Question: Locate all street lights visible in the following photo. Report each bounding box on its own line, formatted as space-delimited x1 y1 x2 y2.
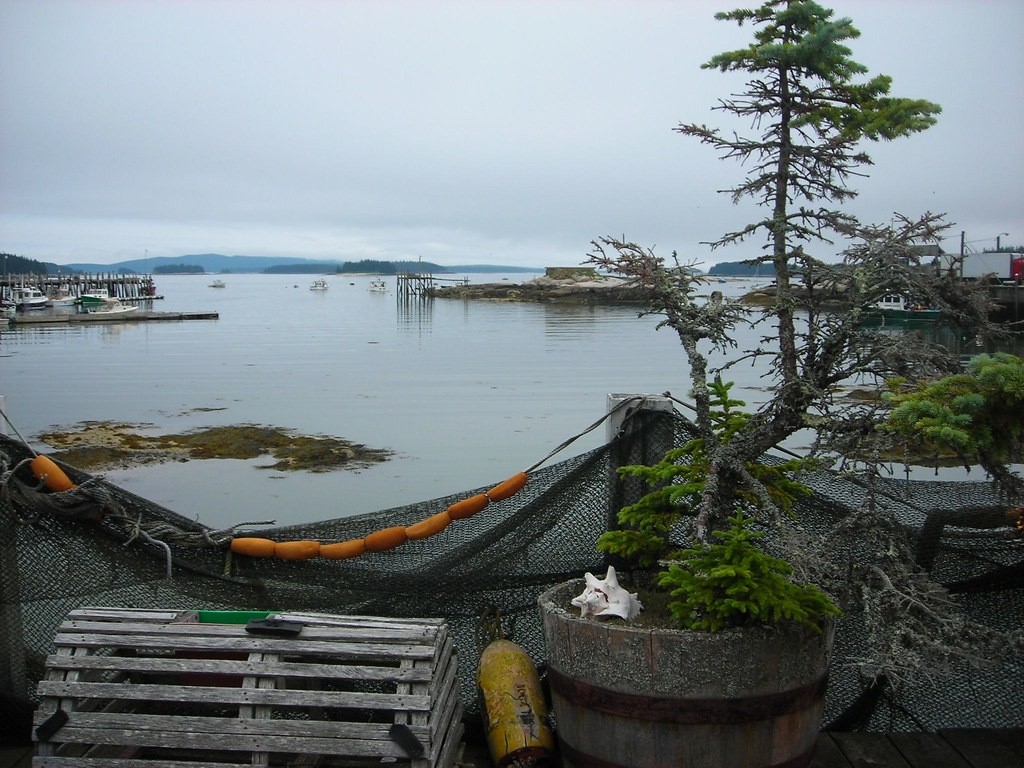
997 232 1010 251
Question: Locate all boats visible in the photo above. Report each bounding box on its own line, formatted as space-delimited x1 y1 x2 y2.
207 279 226 287
369 280 390 292
310 278 330 289
13 278 156 315
859 294 941 322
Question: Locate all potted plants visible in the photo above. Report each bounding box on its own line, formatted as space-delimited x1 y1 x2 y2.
881 355 1024 583
538 1 977 768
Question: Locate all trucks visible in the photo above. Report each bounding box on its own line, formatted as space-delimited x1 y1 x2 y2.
940 251 1024 282
